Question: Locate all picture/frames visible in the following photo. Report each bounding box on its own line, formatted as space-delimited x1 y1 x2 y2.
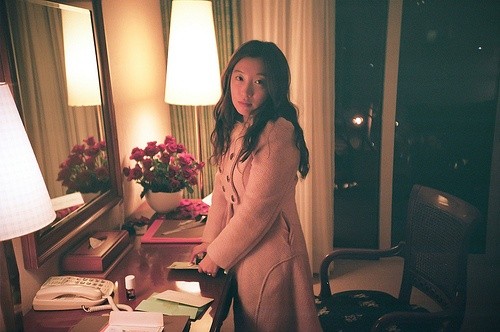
140 215 206 244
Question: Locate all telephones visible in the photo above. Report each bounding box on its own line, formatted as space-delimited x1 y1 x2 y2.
32 274 115 312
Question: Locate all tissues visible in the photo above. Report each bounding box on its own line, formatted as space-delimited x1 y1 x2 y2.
63 230 129 272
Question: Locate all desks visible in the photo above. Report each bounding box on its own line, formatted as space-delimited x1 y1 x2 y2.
23 230 232 332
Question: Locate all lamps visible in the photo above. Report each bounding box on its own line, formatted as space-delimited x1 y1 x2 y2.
164 0 223 200
61 8 104 142
0 82 56 332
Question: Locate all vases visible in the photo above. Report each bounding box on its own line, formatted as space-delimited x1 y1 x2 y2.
145 189 183 214
81 192 100 203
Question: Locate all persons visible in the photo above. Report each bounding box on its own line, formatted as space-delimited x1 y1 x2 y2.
190 41 322 332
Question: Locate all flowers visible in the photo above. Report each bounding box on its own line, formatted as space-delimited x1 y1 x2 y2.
55 136 109 194
123 136 205 200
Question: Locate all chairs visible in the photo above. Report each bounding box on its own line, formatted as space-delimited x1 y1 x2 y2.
314 183 482 332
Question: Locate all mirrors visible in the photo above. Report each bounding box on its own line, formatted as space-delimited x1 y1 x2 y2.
0 0 122 271
